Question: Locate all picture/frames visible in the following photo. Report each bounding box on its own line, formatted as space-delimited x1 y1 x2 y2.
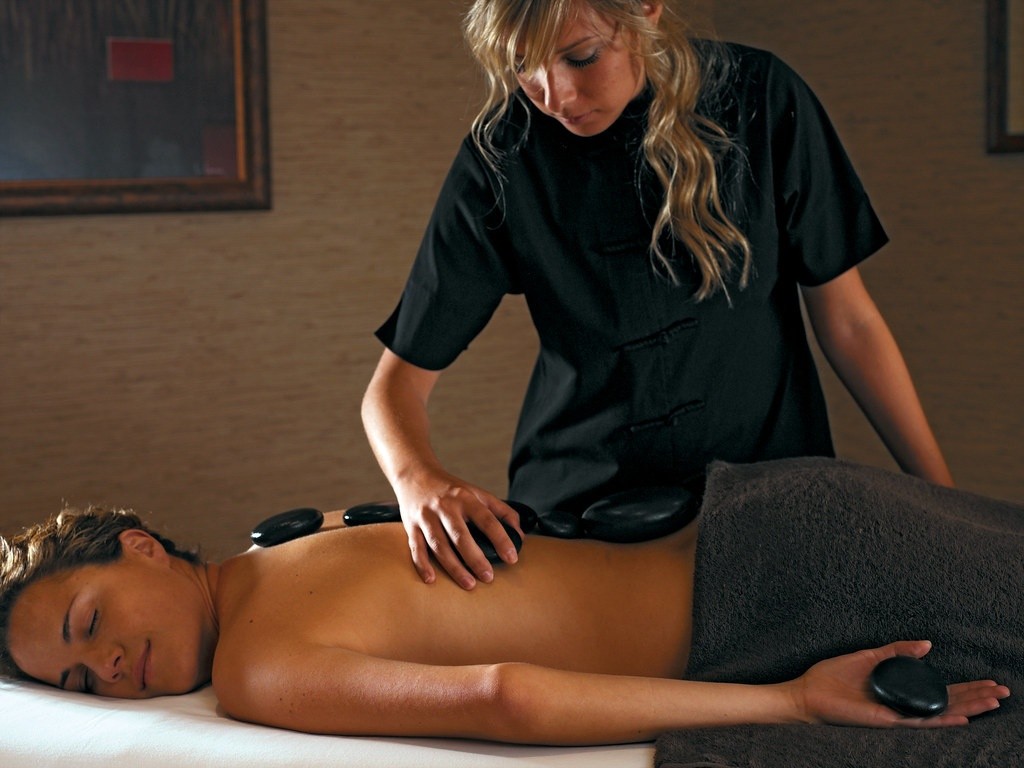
984 0 1024 157
0 0 272 217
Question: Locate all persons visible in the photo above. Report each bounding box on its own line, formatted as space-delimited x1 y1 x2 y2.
360 1 952 589
0 458 1024 747
